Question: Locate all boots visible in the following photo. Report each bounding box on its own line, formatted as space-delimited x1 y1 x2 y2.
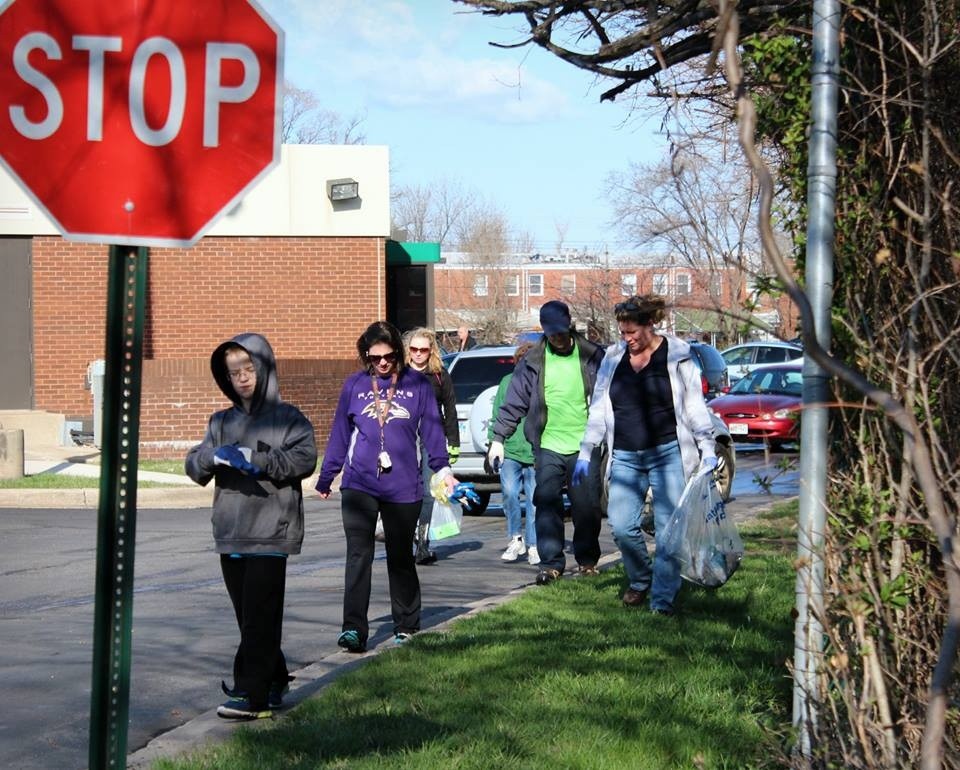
416 523 436 564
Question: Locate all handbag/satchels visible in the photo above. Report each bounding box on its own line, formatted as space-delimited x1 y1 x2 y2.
660 465 744 586
429 467 463 540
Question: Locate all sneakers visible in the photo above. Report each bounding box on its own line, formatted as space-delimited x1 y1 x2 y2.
536 567 562 584
501 534 527 562
395 630 416 644
579 564 596 577
268 693 284 708
528 546 541 566
216 695 274 719
336 630 367 649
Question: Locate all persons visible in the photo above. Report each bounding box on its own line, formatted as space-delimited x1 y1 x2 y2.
488 299 605 584
400 326 461 565
315 321 462 654
484 342 544 565
185 331 318 719
572 292 720 619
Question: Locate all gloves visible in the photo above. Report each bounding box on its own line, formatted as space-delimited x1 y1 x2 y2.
234 447 252 475
701 458 718 469
213 445 260 475
484 443 499 476
448 446 460 465
447 482 481 510
571 460 589 486
488 442 504 468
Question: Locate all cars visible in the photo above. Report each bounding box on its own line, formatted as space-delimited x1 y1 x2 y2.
706 365 804 450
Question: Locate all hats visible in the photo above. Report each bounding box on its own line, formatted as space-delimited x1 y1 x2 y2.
540 301 570 337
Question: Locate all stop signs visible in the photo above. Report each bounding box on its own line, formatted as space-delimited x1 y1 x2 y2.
0 0 285 248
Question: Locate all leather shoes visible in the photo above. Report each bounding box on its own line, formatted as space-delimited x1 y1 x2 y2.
623 587 647 606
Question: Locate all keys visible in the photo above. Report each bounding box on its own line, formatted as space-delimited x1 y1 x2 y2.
376 459 383 480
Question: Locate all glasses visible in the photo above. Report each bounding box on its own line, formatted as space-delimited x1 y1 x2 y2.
410 347 432 353
366 350 399 365
227 366 256 381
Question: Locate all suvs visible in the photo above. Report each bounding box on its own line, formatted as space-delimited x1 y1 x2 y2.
441 345 736 524
687 339 730 402
720 342 805 378
